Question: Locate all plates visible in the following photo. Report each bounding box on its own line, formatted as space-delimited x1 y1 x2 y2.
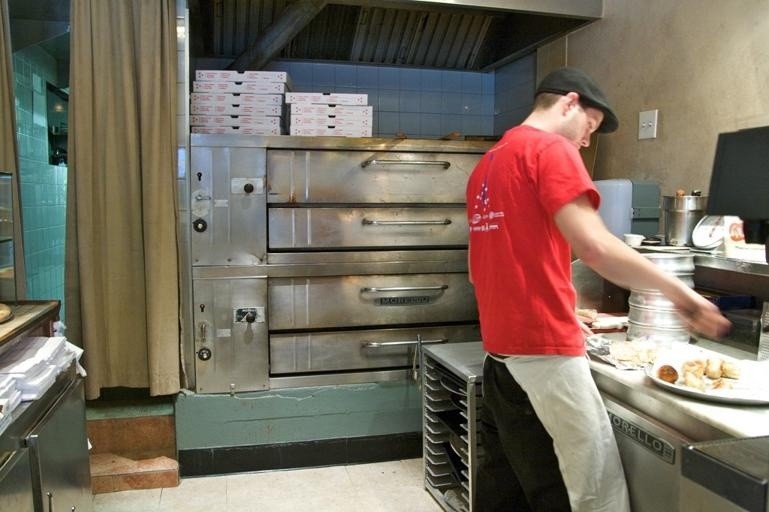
644 351 768 406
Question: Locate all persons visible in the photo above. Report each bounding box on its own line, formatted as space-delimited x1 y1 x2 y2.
466 67 731 512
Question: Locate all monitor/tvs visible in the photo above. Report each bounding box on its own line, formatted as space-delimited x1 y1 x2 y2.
706 126 769 222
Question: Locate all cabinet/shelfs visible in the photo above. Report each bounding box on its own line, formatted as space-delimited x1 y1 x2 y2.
678 436 769 512
0 297 93 512
422 339 484 512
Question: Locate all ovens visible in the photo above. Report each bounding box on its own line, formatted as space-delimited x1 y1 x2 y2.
190 270 486 394
191 133 504 268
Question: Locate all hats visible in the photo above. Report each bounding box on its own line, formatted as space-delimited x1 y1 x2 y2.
533 67 618 134
